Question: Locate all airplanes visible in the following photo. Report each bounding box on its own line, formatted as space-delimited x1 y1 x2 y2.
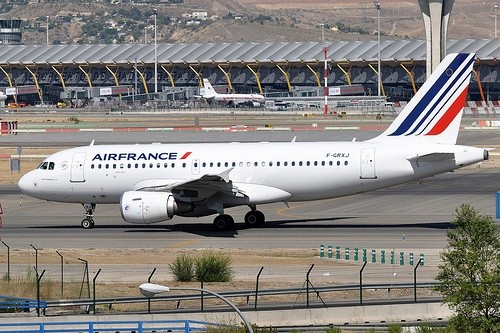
200 76 266 108
19 53 488 231
